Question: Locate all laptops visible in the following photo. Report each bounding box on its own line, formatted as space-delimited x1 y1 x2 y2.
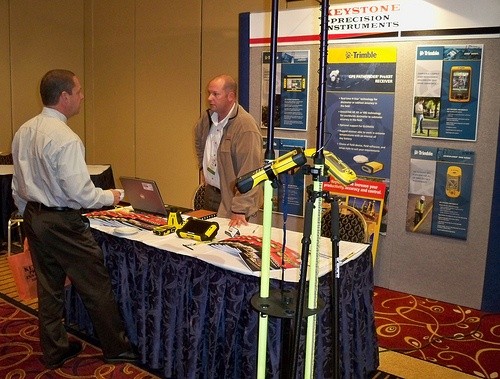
119 176 193 217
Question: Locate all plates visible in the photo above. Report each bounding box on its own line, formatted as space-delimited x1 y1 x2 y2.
115 227 139 235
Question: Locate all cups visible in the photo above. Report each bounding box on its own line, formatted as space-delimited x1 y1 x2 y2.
116 189 124 200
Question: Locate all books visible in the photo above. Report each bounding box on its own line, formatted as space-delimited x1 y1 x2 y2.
186 209 217 220
84 211 168 233
209 235 308 272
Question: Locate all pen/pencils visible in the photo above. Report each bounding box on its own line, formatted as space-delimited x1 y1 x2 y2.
342 253 354 263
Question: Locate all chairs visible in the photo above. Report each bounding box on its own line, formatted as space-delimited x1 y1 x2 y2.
318 205 368 245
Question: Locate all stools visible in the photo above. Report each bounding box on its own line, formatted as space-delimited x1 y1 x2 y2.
8 211 24 258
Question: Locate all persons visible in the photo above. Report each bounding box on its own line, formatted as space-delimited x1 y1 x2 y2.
415 99 425 134
12 70 143 368
194 74 263 228
453 76 465 88
415 196 425 225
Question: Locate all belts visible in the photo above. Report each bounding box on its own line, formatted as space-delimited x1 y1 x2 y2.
209 184 221 193
27 201 73 212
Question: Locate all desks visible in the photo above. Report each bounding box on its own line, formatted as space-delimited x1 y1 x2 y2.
0 164 112 246
65 206 380 379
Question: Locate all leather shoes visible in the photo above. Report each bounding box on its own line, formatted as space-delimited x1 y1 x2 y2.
104 346 140 362
46 341 82 367
